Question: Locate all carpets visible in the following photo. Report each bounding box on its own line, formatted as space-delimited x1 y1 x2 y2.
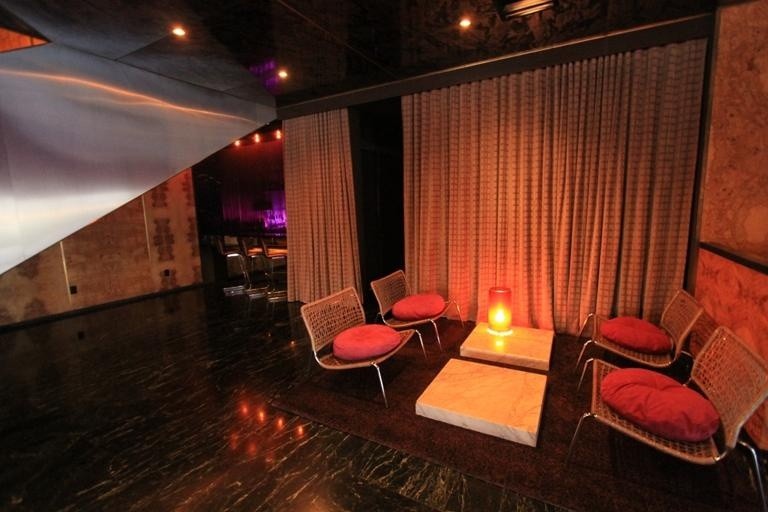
270 318 767 512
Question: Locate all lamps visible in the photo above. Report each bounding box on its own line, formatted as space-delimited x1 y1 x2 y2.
487 287 512 334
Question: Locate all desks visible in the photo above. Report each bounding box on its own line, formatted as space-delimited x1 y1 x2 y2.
415 322 554 448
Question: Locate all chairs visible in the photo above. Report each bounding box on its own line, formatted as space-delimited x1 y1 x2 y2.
212 237 287 303
300 270 465 408
